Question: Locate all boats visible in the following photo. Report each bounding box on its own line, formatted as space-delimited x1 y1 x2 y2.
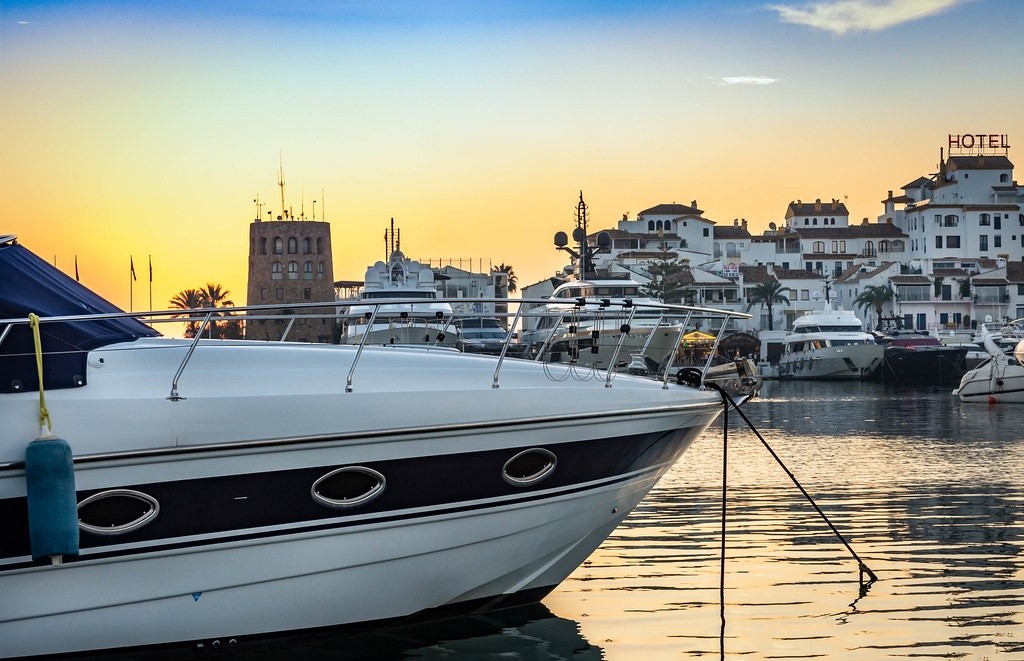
667 330 756 388
952 289 1024 405
522 189 683 374
0 236 748 661
970 290 1020 351
877 309 970 384
752 264 885 382
338 218 459 352
453 315 513 352
